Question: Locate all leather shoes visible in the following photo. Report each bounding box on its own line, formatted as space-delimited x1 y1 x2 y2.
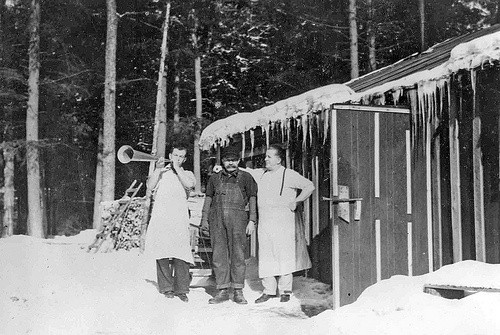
208 290 229 304
280 294 289 302
234 290 248 304
164 291 174 298
177 294 189 302
255 295 276 303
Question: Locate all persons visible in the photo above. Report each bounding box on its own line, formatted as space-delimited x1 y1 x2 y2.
213 143 315 303
145 146 197 302
201 146 258 304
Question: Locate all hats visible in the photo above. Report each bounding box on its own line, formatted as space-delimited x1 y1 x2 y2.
221 146 240 159
